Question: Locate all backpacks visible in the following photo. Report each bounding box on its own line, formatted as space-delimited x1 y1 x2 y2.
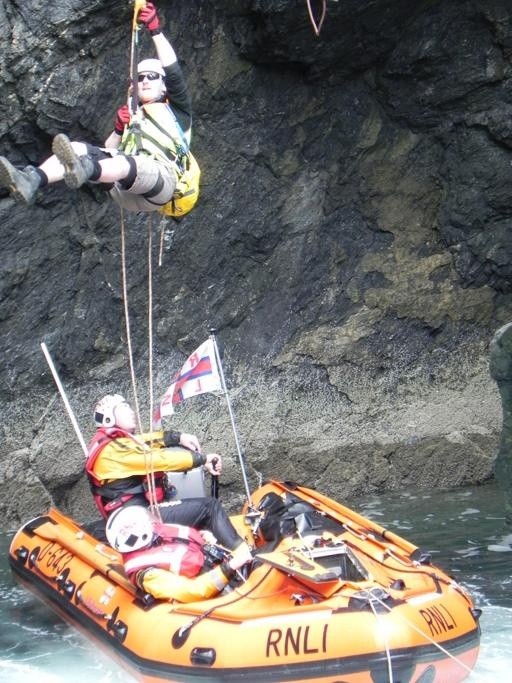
157 150 200 216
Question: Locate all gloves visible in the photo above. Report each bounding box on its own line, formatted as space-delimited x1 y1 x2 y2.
137 3 161 36
114 106 131 135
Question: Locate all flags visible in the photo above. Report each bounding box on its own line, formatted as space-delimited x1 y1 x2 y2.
153 336 218 422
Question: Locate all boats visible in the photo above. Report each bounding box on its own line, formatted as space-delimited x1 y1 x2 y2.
9 477 482 683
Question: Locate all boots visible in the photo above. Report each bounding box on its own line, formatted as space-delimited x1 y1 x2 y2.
1 156 41 205
51 134 94 189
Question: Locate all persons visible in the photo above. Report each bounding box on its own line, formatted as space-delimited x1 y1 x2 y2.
0 1 194 212
104 503 255 606
82 391 250 550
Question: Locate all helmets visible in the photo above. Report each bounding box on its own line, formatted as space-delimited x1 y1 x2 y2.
137 59 166 76
94 394 129 427
106 504 154 554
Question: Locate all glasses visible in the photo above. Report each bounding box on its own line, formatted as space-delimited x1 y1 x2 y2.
139 72 160 82
